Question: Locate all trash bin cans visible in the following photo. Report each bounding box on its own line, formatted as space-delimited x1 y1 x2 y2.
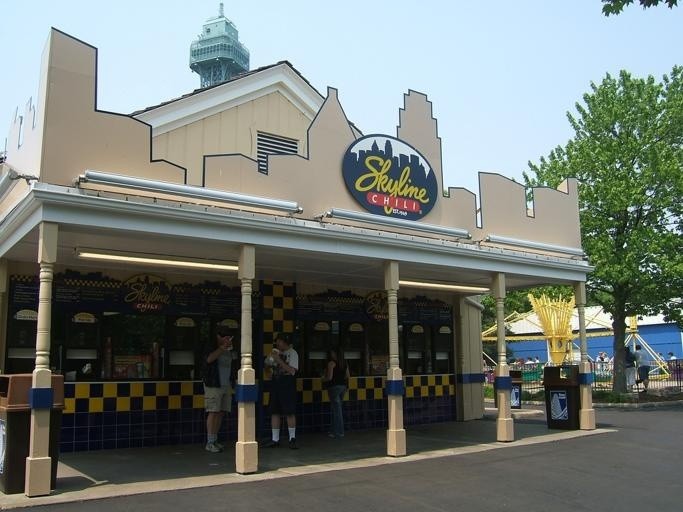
0 374 65 495
544 365 581 430
509 370 522 409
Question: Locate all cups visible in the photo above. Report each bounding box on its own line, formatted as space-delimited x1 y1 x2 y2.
136 362 144 378
270 348 279 361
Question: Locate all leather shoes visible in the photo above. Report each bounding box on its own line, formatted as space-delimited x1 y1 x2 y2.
262 440 280 449
205 441 224 452
288 438 298 450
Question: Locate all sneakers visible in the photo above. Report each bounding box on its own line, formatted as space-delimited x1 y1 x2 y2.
328 433 345 439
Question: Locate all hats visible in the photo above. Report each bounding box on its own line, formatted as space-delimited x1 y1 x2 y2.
215 326 233 336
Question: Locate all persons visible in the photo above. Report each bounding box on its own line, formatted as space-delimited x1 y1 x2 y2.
625 345 650 394
260 332 299 449
525 356 540 364
658 351 677 360
597 352 609 362
323 345 351 439
199 325 238 453
